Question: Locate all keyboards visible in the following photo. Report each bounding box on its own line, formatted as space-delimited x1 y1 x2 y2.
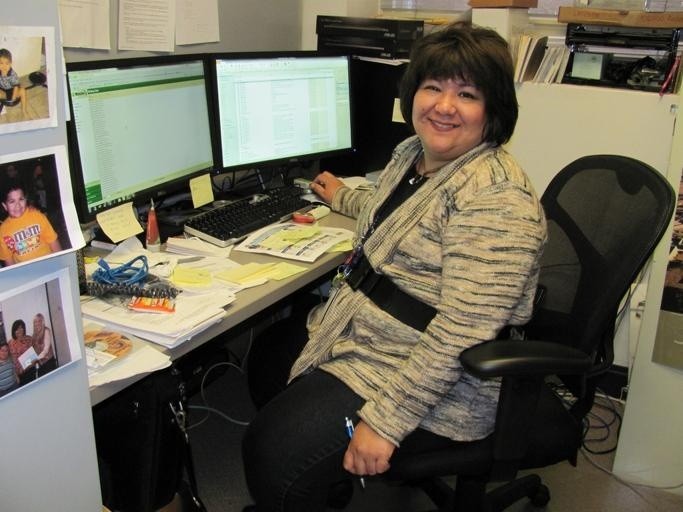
183 185 313 248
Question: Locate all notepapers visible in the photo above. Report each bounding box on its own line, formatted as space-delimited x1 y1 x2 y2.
95 201 145 243
189 173 214 209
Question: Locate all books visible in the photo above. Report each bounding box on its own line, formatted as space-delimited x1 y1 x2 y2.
508 32 570 84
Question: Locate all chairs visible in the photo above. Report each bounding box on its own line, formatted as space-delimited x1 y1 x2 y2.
357 155 675 508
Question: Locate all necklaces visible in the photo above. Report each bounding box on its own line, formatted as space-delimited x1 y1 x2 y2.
332 150 448 288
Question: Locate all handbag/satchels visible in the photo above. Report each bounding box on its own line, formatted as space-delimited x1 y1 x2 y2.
92 365 205 512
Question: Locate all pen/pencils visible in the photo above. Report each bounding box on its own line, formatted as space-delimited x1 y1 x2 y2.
344 416 367 491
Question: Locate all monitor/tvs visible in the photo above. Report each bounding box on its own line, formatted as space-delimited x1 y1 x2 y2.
210 49 357 174
66 53 220 223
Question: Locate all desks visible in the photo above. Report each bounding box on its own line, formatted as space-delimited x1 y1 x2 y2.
43 167 434 407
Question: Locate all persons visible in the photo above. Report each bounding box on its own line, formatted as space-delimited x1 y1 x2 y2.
0 48 28 116
31 313 57 378
0 343 20 400
0 185 62 267
8 319 36 386
240 21 548 511
